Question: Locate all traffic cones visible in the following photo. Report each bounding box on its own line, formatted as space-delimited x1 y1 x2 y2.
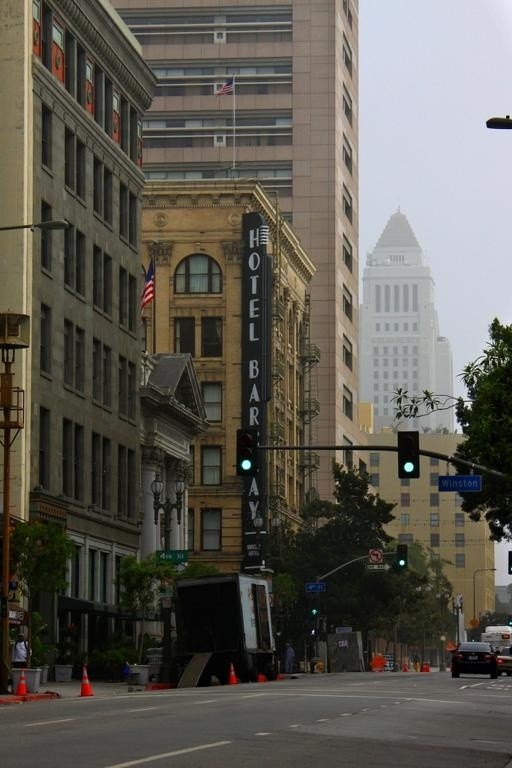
229 662 237 684
81 667 93 696
15 670 27 695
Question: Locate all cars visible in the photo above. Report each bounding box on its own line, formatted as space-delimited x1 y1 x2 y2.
452 641 498 679
497 645 512 676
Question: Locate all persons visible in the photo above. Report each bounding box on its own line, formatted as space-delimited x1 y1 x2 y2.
10 633 29 669
283 642 296 674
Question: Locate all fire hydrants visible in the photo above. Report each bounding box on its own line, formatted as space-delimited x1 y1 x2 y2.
414 661 419 671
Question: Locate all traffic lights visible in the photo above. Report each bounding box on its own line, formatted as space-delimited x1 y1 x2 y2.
397 544 407 568
235 428 259 477
308 607 318 636
398 430 421 480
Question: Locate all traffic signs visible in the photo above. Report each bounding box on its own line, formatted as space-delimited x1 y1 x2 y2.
305 583 326 592
438 475 482 492
156 549 187 563
365 562 391 573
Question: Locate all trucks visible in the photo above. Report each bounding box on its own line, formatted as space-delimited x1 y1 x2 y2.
481 626 512 648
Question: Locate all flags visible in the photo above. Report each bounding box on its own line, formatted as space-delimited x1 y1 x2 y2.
140 259 154 315
214 77 233 97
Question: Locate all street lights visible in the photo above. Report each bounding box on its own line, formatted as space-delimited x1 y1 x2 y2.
150 472 186 679
473 567 496 620
452 593 463 646
0 309 30 694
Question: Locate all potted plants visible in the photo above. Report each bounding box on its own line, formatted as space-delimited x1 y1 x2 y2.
10 516 80 695
114 551 179 687
